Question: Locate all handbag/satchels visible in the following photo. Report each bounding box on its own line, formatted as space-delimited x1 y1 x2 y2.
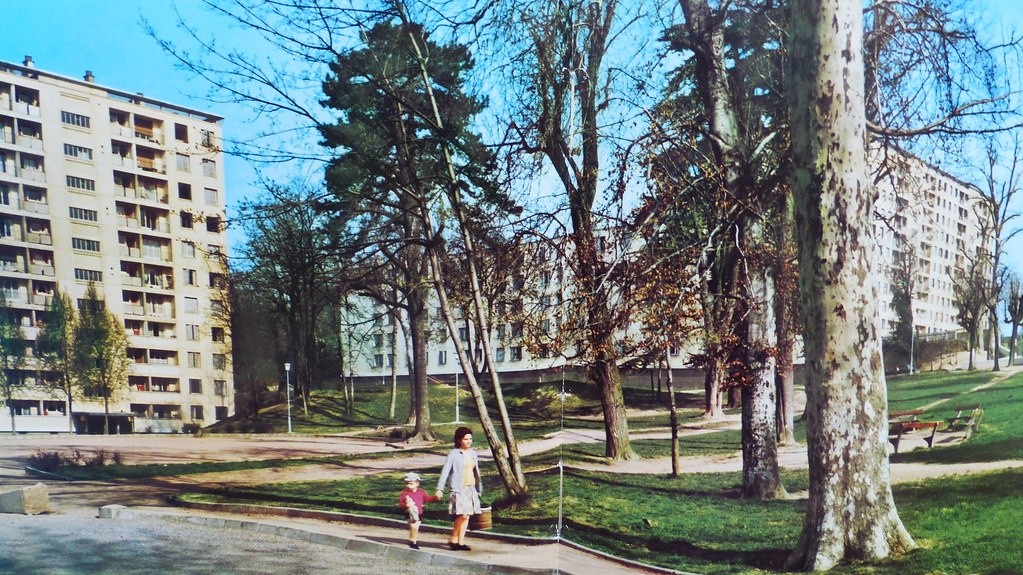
468 495 492 530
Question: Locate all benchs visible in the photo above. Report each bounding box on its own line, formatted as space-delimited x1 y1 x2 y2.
888 402 982 455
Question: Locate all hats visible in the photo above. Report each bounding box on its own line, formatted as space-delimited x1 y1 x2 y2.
404 473 421 481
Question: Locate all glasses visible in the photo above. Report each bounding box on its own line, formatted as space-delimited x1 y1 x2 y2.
405 482 414 485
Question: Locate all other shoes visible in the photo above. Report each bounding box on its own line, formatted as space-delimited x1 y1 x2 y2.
448 541 463 550
410 543 419 549
456 543 471 551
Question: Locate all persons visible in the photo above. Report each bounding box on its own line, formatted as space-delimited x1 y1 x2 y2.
398 473 440 550
436 426 483 551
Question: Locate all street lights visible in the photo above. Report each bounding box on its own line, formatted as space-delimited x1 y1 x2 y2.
453 350 461 420
284 363 292 431
